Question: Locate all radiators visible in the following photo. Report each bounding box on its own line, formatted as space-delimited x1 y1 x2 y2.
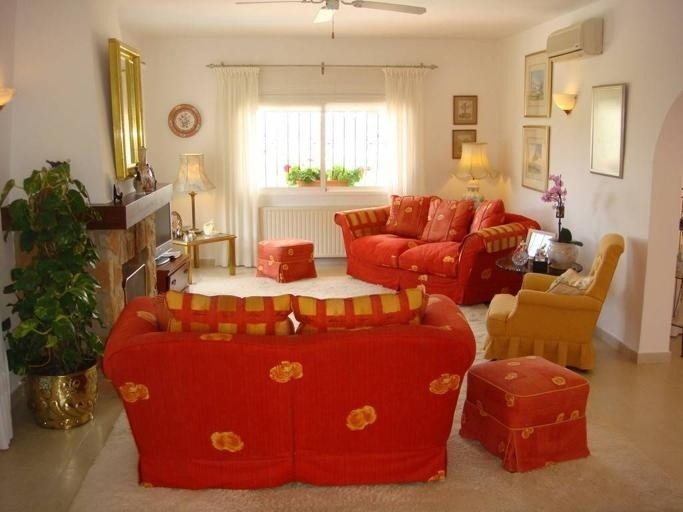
259 204 384 258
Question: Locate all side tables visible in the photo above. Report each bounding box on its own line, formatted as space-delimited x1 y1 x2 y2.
496 255 584 274
173 231 238 284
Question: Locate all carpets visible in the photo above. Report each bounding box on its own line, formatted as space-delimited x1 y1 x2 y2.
69 274 683 512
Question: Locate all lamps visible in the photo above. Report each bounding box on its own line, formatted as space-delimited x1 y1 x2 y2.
173 152 216 233
0 87 15 110
450 142 496 210
552 94 575 115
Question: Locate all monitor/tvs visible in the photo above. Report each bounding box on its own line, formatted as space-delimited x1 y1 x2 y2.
155 203 172 266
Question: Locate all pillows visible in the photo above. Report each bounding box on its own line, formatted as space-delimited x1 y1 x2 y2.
545 268 594 296
166 284 430 335
387 195 504 242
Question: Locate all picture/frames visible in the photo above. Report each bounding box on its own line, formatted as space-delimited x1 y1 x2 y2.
590 83 626 179
525 228 557 262
524 49 552 117
453 95 478 125
521 125 549 192
108 38 145 182
452 130 477 159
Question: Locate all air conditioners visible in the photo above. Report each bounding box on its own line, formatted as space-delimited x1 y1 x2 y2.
546 16 603 62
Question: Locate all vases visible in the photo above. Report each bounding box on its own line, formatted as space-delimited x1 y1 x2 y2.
556 206 564 218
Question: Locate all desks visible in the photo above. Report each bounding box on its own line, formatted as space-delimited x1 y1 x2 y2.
154 254 192 294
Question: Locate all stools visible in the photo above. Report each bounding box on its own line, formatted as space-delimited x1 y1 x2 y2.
459 356 591 472
256 239 318 284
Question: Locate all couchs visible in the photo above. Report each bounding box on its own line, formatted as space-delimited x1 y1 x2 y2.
101 296 477 489
334 207 540 305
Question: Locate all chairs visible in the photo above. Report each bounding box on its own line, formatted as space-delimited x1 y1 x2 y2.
483 233 624 371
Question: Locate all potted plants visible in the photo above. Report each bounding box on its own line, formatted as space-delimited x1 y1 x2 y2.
286 165 365 187
0 161 106 430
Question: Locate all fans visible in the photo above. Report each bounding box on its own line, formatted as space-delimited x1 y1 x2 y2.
235 0 428 23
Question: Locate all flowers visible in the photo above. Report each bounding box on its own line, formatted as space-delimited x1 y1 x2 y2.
539 173 568 209
